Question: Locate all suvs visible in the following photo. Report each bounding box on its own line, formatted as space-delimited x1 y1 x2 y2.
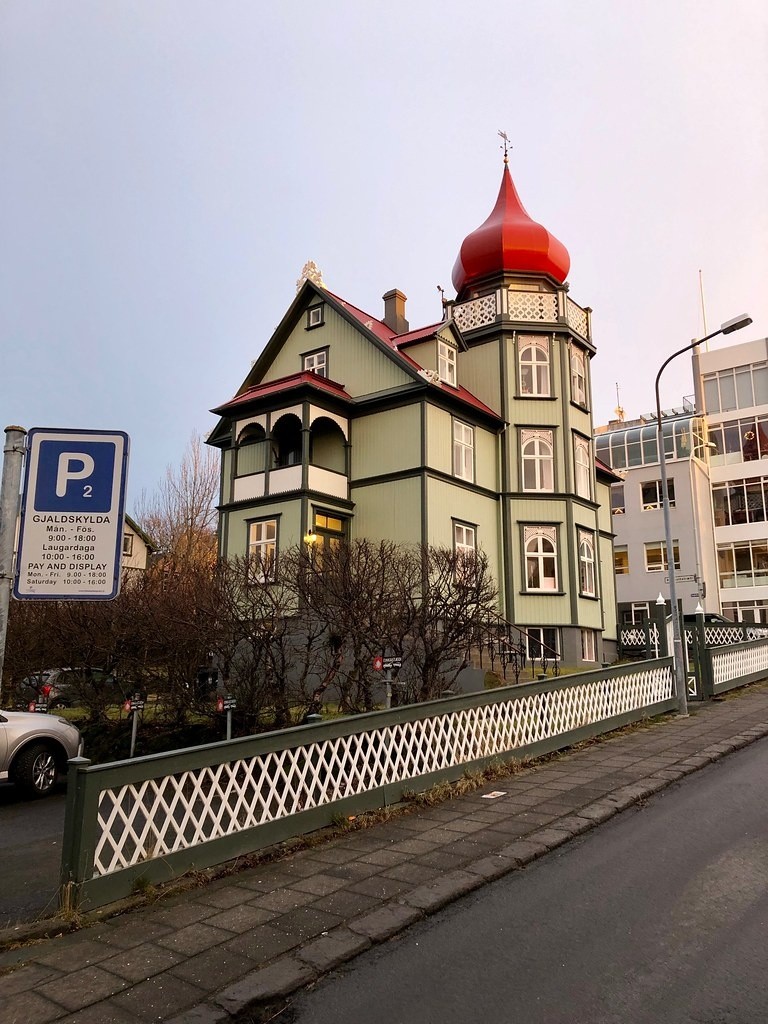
14 667 148 712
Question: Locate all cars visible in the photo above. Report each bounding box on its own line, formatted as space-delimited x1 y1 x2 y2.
0 709 84 797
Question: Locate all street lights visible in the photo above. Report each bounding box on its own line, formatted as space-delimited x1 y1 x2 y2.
654 314 754 717
688 439 716 611
